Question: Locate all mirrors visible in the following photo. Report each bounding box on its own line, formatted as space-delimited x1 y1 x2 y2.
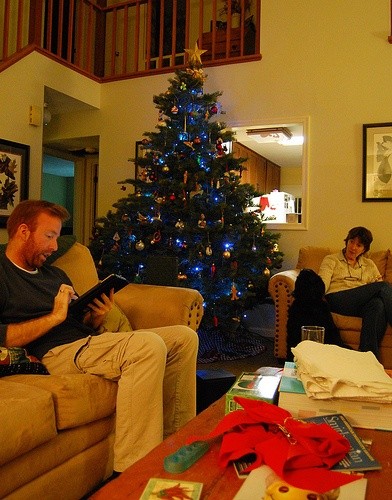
224 116 310 231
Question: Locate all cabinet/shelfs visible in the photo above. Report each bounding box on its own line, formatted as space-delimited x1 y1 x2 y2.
232 142 280 195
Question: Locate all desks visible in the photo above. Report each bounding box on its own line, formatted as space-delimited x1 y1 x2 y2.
88 365 392 500
195 26 253 61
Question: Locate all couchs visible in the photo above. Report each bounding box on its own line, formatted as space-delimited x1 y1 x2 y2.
267 246 392 371
0 238 203 500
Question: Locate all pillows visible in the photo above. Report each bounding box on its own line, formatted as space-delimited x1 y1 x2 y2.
0 347 50 378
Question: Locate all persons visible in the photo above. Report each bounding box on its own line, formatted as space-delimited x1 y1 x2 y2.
0 200 199 478
316 225 392 361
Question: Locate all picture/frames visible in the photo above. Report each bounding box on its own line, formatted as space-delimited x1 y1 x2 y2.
362 122 392 203
0 139 30 227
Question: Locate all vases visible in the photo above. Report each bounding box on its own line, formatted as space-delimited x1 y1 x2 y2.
230 13 241 28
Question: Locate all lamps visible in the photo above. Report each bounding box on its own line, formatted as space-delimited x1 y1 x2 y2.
44 101 52 126
244 127 292 145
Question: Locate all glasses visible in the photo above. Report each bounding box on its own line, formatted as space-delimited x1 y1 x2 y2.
344 276 358 281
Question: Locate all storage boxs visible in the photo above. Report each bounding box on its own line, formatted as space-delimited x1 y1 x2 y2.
278 370 392 431
225 371 282 415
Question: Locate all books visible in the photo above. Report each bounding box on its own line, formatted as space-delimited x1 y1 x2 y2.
277 361 392 432
232 414 382 480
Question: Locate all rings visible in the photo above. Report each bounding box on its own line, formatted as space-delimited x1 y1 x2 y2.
59 289 63 292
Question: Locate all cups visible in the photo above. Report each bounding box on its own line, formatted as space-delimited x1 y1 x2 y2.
301 326 325 345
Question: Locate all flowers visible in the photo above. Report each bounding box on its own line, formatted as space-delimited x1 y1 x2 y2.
219 0 251 19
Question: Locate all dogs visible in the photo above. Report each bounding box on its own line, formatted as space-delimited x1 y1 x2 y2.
284 266 352 362
